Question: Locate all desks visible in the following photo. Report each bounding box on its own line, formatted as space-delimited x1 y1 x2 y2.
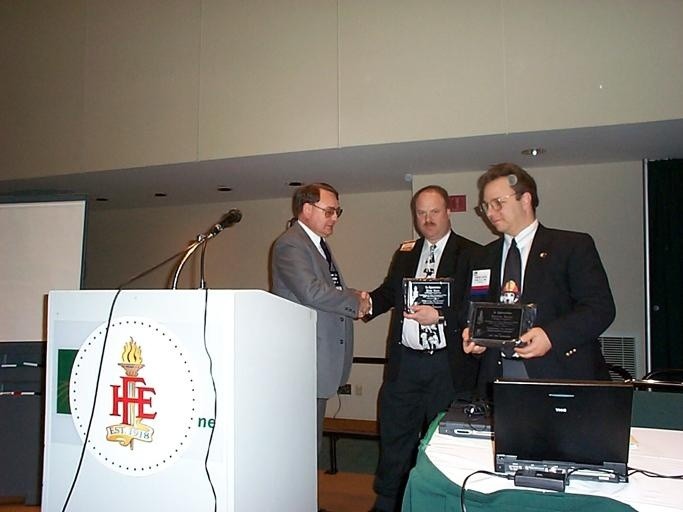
402 391 683 512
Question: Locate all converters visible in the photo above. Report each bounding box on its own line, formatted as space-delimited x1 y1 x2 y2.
514 469 566 492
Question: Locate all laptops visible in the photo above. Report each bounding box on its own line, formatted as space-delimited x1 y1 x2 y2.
439 399 494 438
493 377 634 483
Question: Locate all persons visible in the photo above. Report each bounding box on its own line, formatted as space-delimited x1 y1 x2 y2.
460 163 616 402
354 185 487 512
267 182 369 457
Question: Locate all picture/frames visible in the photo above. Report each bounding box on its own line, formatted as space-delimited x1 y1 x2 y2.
401 277 454 315
467 303 525 347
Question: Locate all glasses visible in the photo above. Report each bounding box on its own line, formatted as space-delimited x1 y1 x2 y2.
310 203 344 219
480 190 524 215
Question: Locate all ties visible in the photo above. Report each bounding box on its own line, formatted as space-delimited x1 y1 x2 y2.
421 244 437 279
502 237 521 290
320 237 342 291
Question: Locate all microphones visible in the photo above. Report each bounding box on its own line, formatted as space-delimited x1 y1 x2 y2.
210 209 241 236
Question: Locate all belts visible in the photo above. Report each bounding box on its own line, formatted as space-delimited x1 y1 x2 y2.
399 343 447 356
